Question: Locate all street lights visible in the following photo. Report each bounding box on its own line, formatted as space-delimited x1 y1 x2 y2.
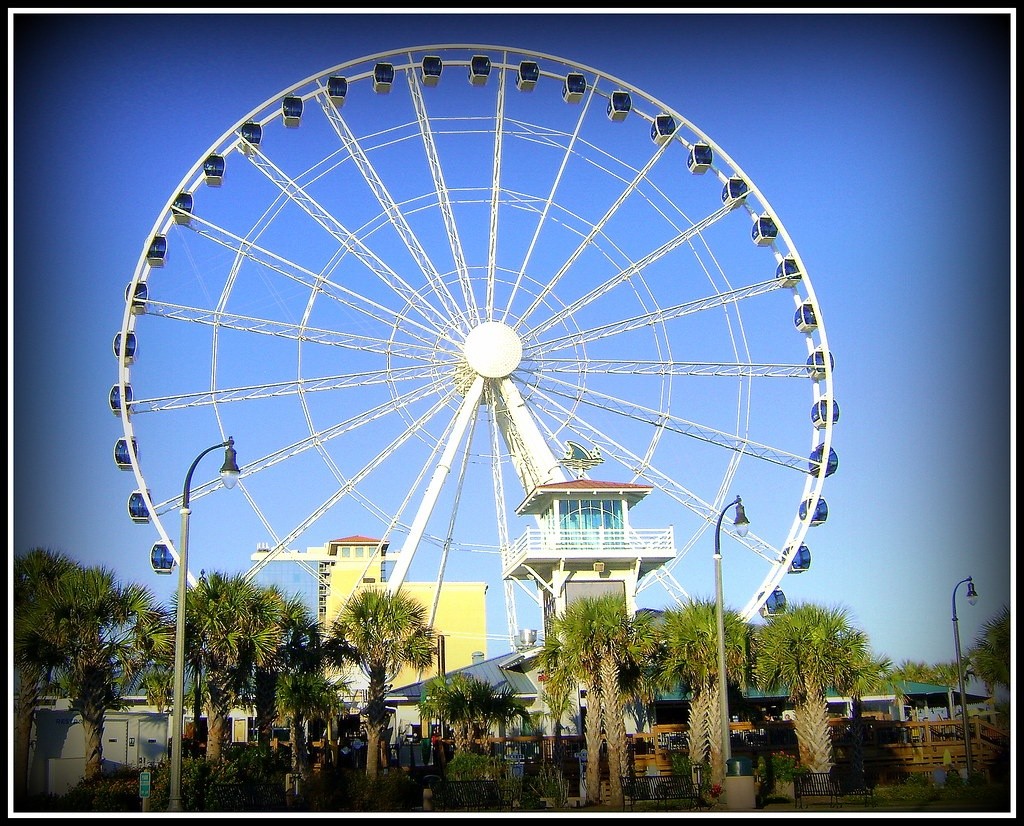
713 495 750 777
165 435 241 812
951 576 978 777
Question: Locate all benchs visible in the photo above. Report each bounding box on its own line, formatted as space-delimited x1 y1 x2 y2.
214 783 297 811
428 779 514 812
619 775 696 811
793 772 878 809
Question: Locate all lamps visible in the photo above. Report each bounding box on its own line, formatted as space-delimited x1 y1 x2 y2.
592 562 605 572
526 573 535 581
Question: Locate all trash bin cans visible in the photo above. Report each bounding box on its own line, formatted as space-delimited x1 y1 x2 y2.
285 774 302 793
725 755 753 776
985 759 1008 785
